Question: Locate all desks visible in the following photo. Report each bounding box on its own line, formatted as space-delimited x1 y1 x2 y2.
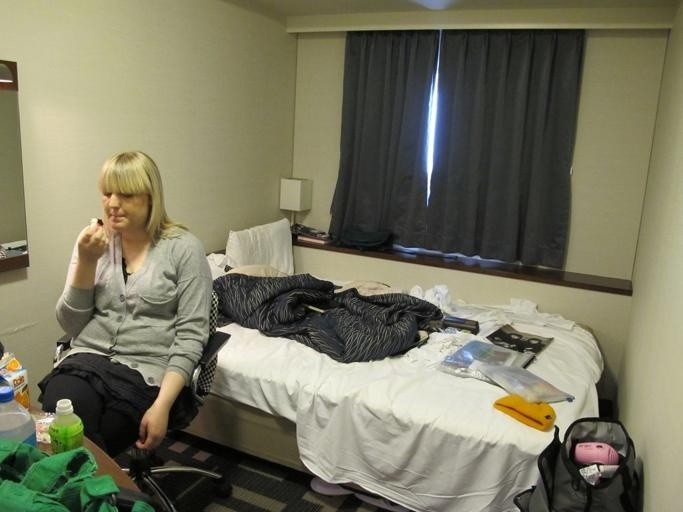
0 399 145 512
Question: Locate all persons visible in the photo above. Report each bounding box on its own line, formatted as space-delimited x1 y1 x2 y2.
492 332 523 351
32 151 212 461
514 337 543 354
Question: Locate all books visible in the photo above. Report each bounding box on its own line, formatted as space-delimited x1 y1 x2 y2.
296 234 336 245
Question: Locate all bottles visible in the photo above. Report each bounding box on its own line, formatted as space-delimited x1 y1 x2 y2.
0 386 36 451
47 398 86 455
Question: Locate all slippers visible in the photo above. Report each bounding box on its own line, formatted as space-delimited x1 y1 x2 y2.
356 492 410 511
310 477 354 497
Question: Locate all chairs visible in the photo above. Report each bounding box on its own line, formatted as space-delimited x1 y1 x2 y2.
52 288 231 512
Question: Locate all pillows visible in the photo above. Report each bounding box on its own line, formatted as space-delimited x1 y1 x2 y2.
220 216 293 287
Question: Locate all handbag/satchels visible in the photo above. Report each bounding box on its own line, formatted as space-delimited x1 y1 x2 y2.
335 229 395 252
1 441 154 512
512 417 638 512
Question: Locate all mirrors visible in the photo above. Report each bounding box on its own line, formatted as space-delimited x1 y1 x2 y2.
0 57 30 272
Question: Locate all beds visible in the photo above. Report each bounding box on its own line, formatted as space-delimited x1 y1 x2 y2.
137 242 603 512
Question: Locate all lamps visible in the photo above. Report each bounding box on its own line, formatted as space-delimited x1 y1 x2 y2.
279 177 313 244
